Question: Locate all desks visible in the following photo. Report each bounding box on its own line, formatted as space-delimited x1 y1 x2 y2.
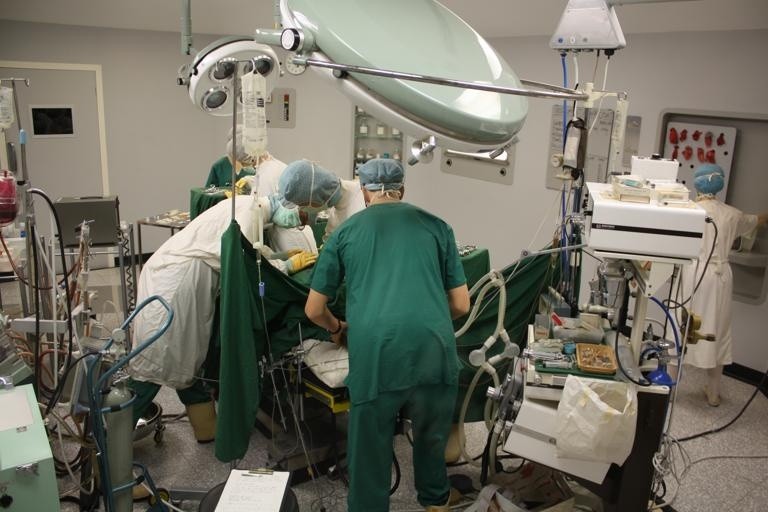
137 209 192 279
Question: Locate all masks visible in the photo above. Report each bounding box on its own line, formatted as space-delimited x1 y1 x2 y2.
301 204 329 215
272 201 302 228
241 164 256 174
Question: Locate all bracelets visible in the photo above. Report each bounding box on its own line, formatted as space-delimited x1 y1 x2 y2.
327 318 342 335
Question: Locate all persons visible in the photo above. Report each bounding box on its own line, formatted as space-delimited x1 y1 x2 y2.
204 124 255 192
683 161 768 407
225 132 287 200
304 156 475 511
275 159 368 252
120 190 320 445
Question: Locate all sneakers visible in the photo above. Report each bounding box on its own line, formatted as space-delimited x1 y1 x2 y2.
422 474 473 507
704 384 721 407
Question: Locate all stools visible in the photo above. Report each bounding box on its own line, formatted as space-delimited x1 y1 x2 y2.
193 477 300 512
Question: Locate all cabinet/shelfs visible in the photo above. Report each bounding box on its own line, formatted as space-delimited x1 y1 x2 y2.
346 101 403 188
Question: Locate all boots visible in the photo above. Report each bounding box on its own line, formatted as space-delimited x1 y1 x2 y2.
184 401 219 445
131 480 153 501
